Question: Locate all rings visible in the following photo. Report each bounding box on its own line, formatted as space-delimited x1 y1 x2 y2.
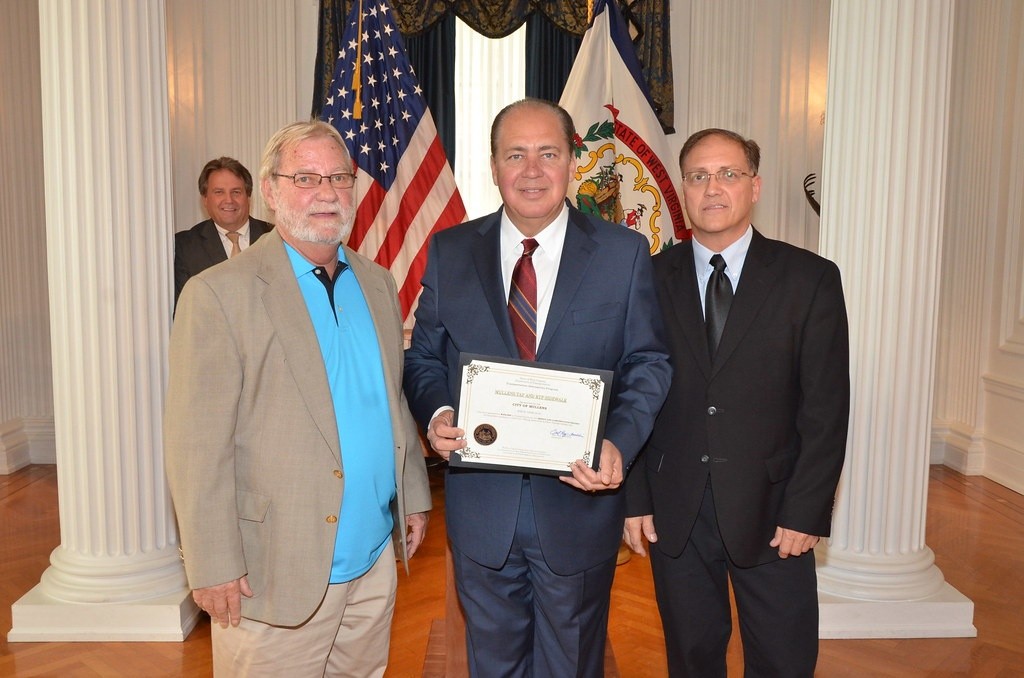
591 490 596 493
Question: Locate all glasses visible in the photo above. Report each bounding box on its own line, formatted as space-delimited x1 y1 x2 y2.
682 169 755 184
273 173 358 190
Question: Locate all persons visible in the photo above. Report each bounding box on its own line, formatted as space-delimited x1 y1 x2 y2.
163 121 432 678
172 156 276 323
403 99 673 678
622 128 850 678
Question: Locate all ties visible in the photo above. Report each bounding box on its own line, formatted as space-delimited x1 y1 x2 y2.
507 238 539 361
705 254 734 363
226 232 241 259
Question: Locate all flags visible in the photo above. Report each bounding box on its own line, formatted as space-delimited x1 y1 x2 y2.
320 0 469 330
558 0 693 256
